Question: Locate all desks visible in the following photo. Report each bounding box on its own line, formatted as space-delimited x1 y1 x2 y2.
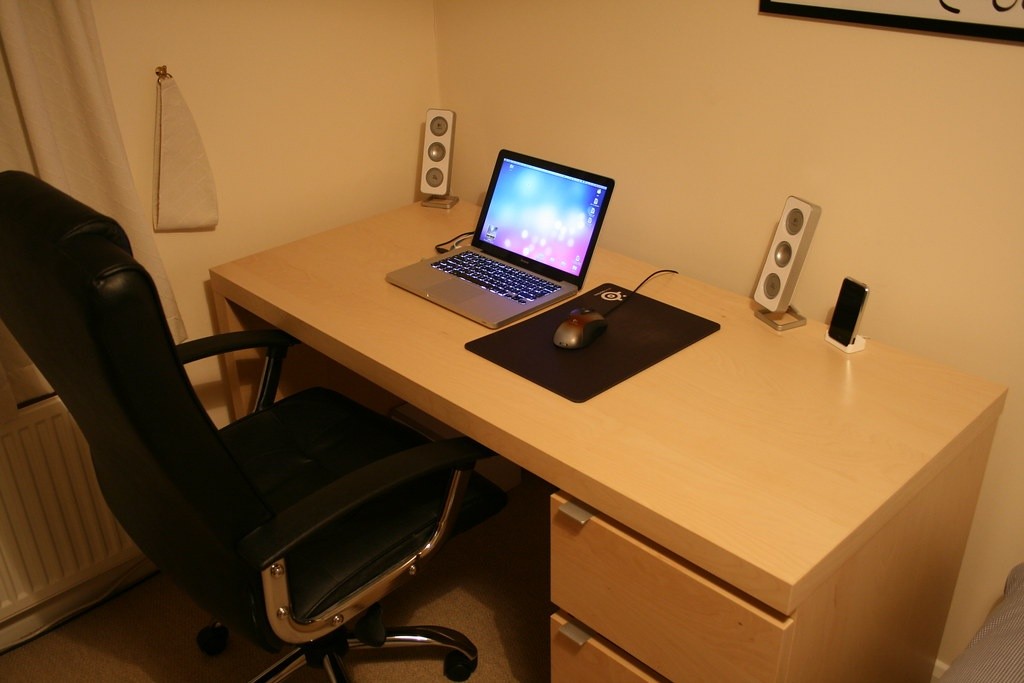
210 194 1007 683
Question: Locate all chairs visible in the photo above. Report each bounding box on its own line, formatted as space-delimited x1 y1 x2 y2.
0 169 510 682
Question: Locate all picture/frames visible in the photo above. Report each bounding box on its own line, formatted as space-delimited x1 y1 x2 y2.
757 0 1024 42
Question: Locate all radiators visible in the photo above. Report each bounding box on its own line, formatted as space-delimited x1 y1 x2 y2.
0 394 152 623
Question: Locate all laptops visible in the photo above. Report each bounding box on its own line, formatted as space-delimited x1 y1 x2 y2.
385 149 616 329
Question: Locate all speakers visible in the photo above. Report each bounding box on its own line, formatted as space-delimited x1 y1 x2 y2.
754 195 821 331
421 108 459 209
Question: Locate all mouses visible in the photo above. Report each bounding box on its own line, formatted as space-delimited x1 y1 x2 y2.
553 307 607 350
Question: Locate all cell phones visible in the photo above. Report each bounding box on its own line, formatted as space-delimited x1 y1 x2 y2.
828 276 870 347
